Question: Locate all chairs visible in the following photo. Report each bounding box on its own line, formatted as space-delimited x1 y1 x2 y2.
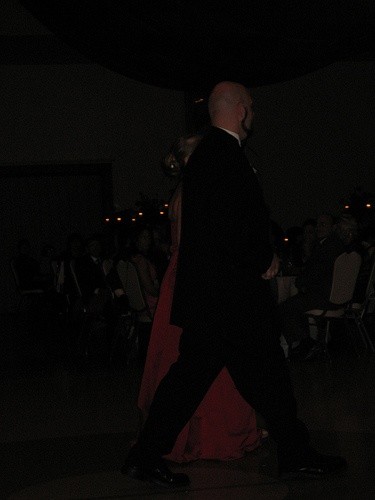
114 259 154 348
304 249 375 370
10 254 57 320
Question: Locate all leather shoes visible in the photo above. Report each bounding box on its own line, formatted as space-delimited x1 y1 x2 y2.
256 436 347 476
121 445 191 489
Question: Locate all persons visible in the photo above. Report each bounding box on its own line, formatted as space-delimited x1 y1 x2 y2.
135 125 271 478
10 208 375 366
120 80 347 489
